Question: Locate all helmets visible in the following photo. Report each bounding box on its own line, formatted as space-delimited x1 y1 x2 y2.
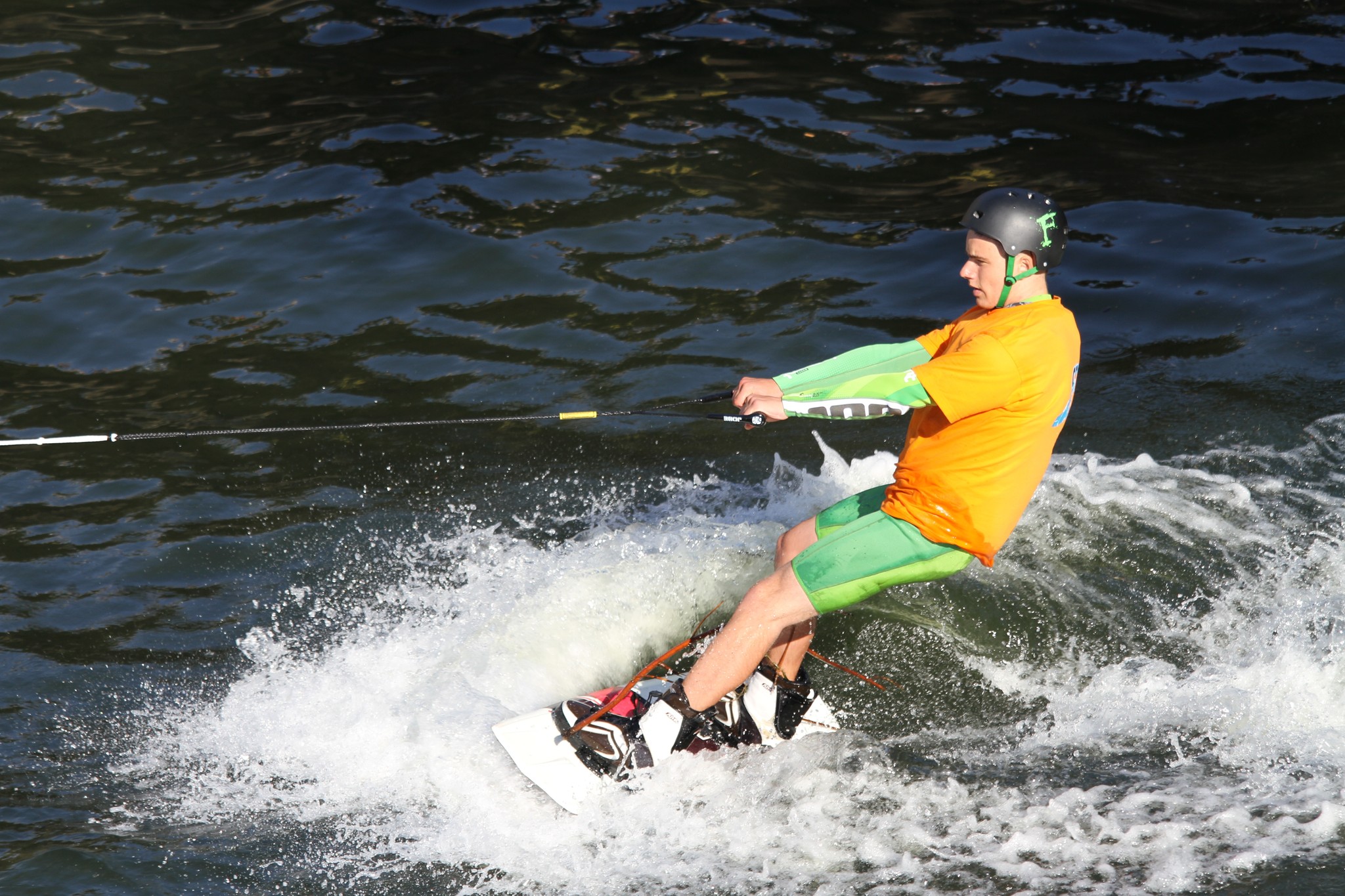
971 186 1067 271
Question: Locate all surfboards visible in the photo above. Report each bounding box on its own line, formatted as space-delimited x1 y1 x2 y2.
491 661 840 816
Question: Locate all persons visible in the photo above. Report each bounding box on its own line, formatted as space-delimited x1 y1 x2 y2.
556 187 1082 795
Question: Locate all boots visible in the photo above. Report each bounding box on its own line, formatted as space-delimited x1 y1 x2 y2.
556 678 720 780
711 655 816 749
